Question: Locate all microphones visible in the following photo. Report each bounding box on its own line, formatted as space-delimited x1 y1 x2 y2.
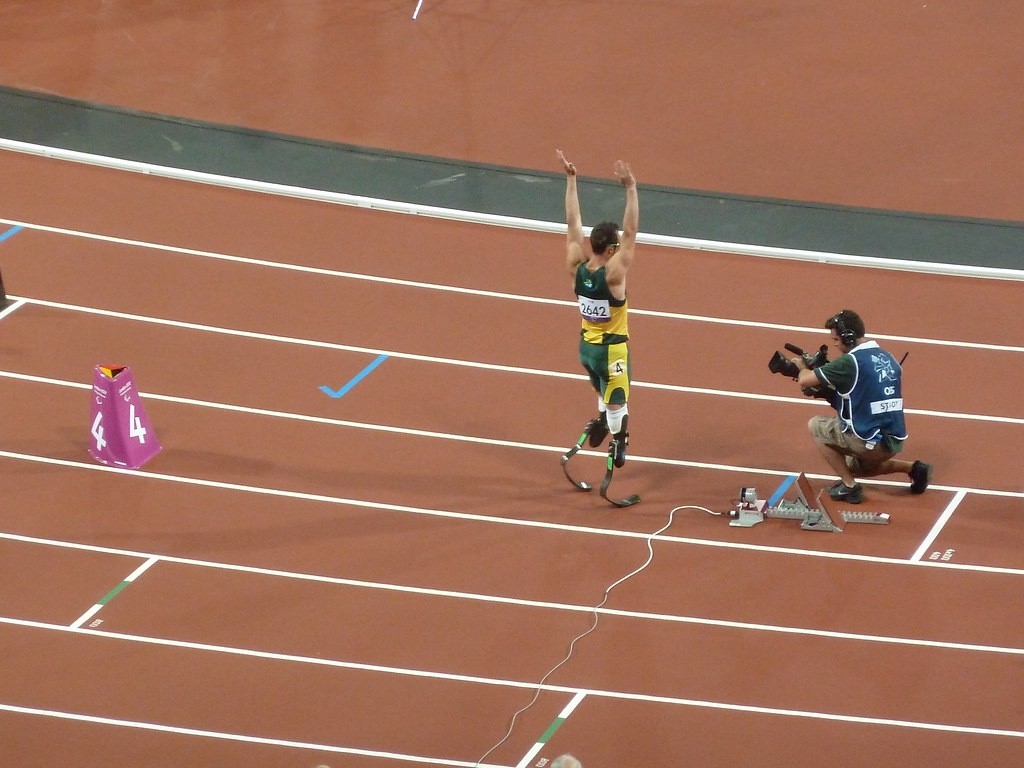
784 343 808 358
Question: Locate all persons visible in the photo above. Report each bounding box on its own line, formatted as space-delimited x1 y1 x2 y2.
790 309 934 506
555 148 639 468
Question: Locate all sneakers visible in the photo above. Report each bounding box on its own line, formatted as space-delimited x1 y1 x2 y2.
908 460 932 494
827 480 864 504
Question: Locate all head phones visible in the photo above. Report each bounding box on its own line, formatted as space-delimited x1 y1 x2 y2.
834 314 858 347
